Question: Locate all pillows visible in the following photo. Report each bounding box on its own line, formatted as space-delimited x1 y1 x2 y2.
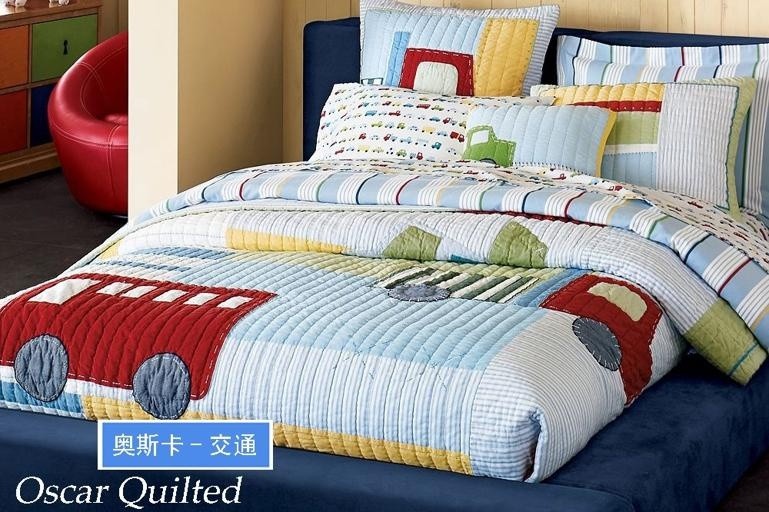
528 76 761 226
311 79 534 175
552 32 769 226
355 1 562 104
457 97 620 186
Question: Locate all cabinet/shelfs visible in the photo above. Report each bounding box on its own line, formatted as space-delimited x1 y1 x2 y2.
0 1 103 187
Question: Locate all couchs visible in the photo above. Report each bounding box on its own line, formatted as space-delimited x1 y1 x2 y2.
44 29 131 216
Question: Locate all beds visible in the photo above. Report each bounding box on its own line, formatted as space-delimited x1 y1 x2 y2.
0 1 769 512
